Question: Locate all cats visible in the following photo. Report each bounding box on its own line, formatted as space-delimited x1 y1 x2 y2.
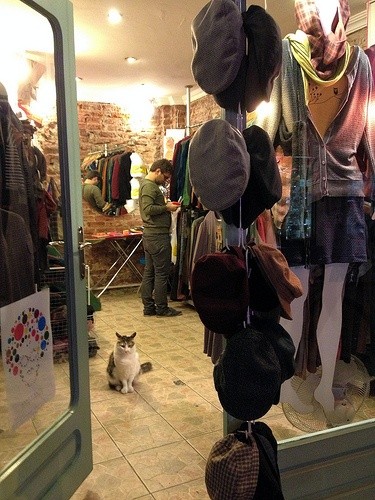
107 331 152 393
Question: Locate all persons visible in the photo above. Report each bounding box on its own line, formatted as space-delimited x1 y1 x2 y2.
82 170 112 213
137 158 183 316
265 32 375 415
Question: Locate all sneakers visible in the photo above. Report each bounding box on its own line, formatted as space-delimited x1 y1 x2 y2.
156 307 183 316
144 307 156 315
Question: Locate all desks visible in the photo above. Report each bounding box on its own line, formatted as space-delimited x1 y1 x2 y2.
86 230 143 298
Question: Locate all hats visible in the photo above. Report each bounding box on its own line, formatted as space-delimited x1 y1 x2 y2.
188 121 250 211
212 339 281 420
190 246 247 334
192 0 246 92
246 4 283 113
247 242 304 320
242 124 283 230
232 420 285 500
246 318 294 383
204 430 259 500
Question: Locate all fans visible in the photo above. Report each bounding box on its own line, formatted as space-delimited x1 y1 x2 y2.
282 356 370 433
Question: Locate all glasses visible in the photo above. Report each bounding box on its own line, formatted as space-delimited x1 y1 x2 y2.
161 170 169 181
